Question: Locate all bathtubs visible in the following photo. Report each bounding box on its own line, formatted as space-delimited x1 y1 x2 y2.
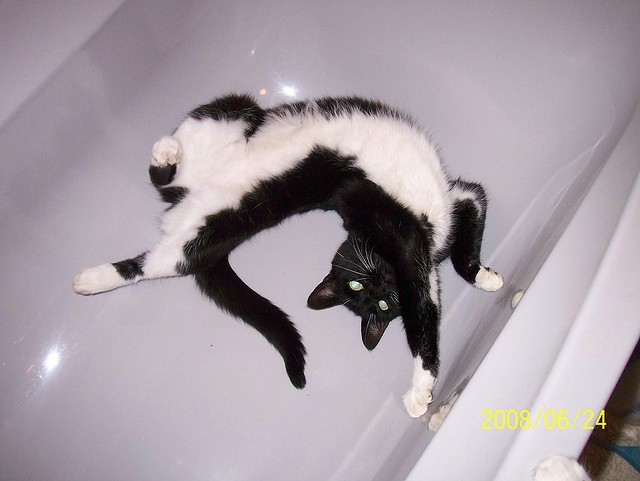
0 1 640 481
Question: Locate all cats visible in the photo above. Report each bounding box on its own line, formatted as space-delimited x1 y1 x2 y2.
72 92 503 418
